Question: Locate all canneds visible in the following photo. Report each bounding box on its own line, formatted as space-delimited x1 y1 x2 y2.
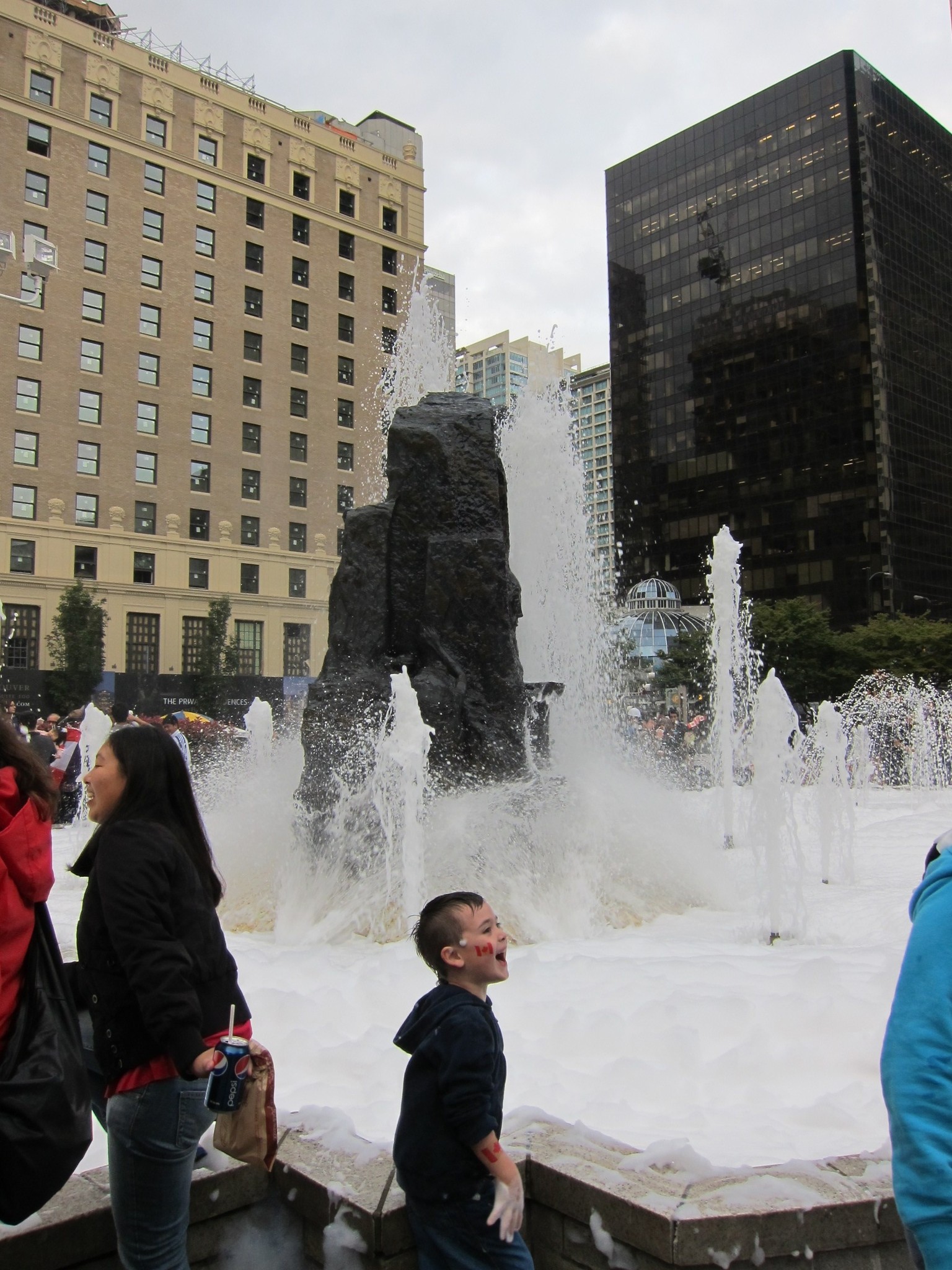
203 1036 249 1114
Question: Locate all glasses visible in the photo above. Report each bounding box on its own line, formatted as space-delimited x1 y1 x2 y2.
47 720 56 725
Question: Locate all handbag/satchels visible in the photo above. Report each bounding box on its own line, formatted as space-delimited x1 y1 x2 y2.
0 904 92 1225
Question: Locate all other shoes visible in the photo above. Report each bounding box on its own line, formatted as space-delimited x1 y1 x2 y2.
193 1144 208 1171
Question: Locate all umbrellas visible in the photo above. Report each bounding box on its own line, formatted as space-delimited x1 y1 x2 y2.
160 709 213 723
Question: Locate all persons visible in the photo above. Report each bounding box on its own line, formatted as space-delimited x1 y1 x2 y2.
626 700 705 757
0 699 64 761
392 892 536 1270
112 703 154 733
0 718 55 1038
64 726 272 1270
163 714 190 770
878 828 952 1270
